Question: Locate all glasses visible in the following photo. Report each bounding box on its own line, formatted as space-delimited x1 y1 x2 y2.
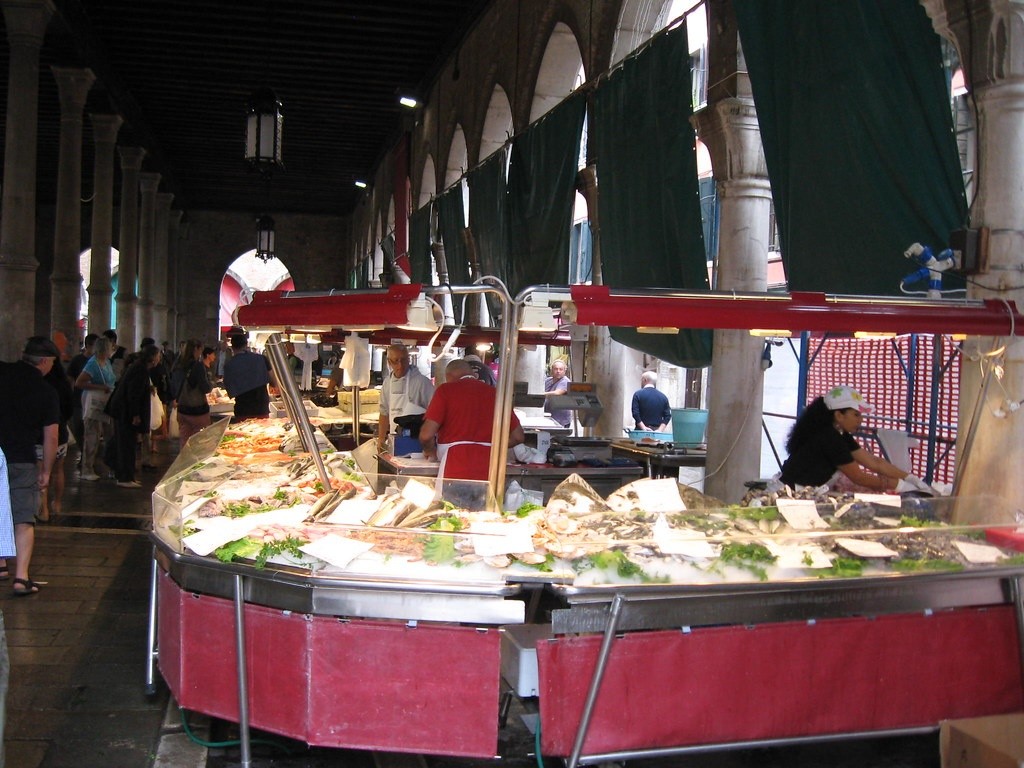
390 359 411 365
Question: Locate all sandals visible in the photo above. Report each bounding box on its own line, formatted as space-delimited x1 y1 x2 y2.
12 578 43 595
0 566 12 580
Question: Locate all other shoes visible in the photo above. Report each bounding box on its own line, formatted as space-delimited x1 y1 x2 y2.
80 472 101 481
116 479 142 488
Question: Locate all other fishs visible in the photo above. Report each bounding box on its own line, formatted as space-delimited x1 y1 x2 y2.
168 383 999 573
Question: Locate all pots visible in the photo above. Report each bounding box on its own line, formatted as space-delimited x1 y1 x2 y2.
623 428 672 442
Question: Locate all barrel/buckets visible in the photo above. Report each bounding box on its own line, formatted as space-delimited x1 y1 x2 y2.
671 408 708 448
395 435 437 456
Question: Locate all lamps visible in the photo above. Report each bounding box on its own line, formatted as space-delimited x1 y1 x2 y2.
395 90 425 109
290 334 306 343
636 326 680 334
397 293 439 332
306 334 322 344
342 324 385 332
351 174 370 189
749 329 792 338
854 332 897 341
247 326 286 333
255 182 277 261
243 0 286 184
291 325 332 333
281 334 289 342
517 305 559 333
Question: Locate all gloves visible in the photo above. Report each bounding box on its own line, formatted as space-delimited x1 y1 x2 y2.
895 474 928 493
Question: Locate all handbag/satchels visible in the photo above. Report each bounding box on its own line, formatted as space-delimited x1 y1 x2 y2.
150 390 164 429
168 408 180 438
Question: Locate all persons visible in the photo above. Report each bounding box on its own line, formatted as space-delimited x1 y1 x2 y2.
465 345 498 385
420 360 526 510
780 386 931 494
285 341 323 378
544 360 573 429
225 333 276 421
67 334 100 465
631 371 671 431
377 344 436 451
36 369 72 525
75 339 116 480
168 340 211 451
200 340 229 384
0 448 16 740
105 331 181 489
0 337 61 595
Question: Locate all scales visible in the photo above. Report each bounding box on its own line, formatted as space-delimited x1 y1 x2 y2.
552 382 612 461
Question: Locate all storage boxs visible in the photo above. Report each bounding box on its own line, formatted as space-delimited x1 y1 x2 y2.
269 400 319 418
206 388 235 412
337 389 380 415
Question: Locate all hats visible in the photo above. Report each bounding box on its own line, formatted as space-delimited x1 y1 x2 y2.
823 386 876 413
22 336 60 357
